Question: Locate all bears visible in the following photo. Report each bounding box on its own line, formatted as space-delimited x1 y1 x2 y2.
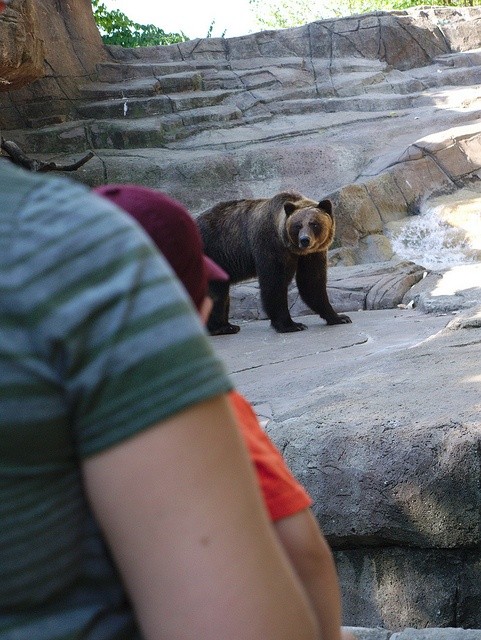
192 192 351 336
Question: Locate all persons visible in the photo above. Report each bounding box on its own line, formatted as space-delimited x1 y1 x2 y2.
83 182 360 639
0 149 327 639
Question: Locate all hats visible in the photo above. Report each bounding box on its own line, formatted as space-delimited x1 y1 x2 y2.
95 185 230 285
